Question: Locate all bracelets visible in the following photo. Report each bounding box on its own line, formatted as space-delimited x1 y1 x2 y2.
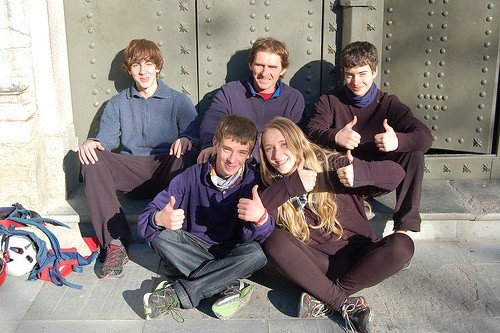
254 208 267 225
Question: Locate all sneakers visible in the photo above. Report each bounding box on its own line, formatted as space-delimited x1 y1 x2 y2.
100 243 129 279
337 296 373 333
211 279 256 320
143 280 184 323
297 292 336 319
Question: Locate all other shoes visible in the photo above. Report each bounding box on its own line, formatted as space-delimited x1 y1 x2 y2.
402 259 411 271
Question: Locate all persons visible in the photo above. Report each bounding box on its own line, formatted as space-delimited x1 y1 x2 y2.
78 39 202 280
304 41 433 271
200 37 306 189
136 114 275 320
258 117 415 333
